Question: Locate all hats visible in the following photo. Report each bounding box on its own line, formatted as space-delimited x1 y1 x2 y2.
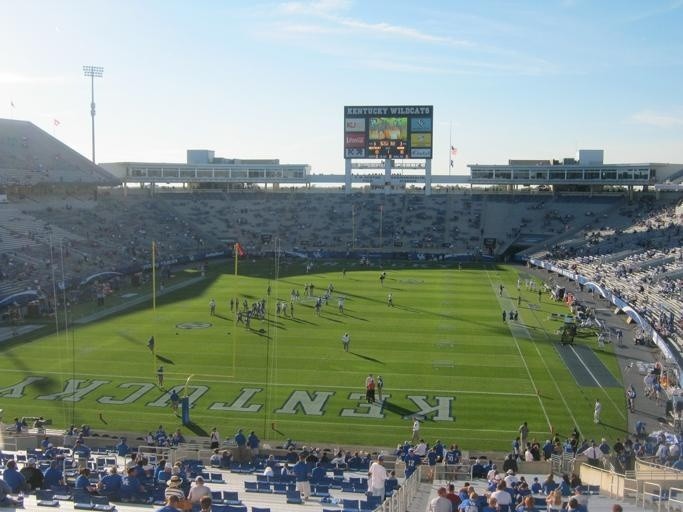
167 476 183 487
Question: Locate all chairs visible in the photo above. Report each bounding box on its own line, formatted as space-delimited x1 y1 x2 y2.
2 193 683 386
0 437 683 512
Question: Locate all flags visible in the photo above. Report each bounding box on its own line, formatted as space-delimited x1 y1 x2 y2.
451 160 454 167
451 145 457 156
54 119 60 127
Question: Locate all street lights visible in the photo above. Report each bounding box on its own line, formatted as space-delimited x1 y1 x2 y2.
83 65 103 201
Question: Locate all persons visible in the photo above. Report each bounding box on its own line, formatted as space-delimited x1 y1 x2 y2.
1 174 215 335
499 173 683 511
170 389 180 413
158 366 164 387
342 333 350 352
377 375 384 400
276 282 345 318
366 374 376 403
148 336 154 353
388 293 393 307
212 173 544 278
380 272 386 286
231 284 272 329
1 416 511 511
